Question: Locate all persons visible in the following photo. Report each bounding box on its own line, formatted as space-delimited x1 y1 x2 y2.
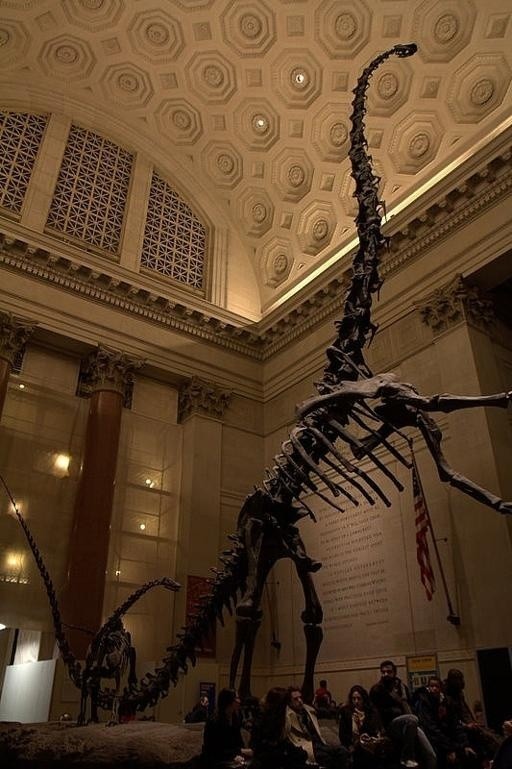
315 679 332 700
338 660 512 769
201 686 254 769
285 686 352 769
247 686 310 769
184 693 212 723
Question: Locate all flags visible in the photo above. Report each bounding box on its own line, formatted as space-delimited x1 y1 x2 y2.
409 456 438 602
